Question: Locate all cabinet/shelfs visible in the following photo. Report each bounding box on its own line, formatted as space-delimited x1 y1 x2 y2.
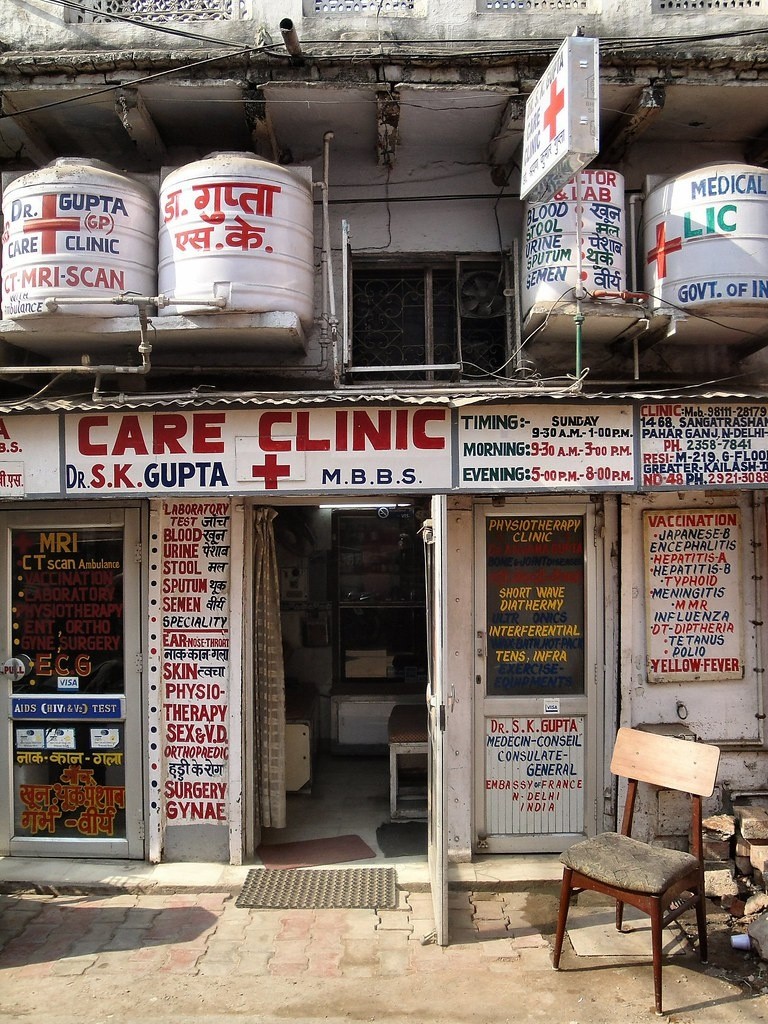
329 508 431 695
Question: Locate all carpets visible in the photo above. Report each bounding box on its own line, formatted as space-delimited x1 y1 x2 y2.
376 821 428 858
235 867 398 910
256 834 377 869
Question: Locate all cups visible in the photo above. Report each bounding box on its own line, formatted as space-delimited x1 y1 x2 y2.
730 934 750 950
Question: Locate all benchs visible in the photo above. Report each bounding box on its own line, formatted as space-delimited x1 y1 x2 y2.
282 679 318 795
387 704 429 821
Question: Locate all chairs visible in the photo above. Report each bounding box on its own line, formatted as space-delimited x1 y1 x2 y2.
552 726 720 1017
